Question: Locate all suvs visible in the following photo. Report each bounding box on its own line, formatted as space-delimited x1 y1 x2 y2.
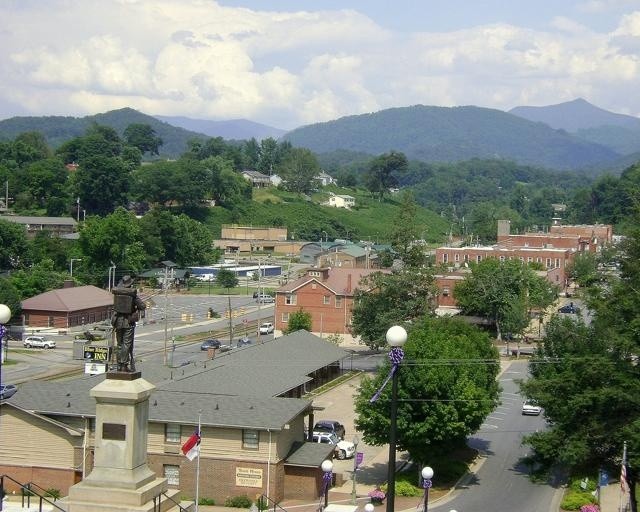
307 431 355 460
23 336 56 349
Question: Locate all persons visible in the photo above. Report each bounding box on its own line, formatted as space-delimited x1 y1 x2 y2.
112 275 146 372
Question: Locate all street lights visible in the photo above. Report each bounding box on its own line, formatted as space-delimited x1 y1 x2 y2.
365 503 374 512
71 259 82 276
386 325 407 512
422 466 434 512
0 304 11 387
108 267 117 292
321 460 333 507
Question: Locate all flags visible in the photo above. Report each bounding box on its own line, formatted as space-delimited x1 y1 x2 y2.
181 433 201 461
620 447 626 495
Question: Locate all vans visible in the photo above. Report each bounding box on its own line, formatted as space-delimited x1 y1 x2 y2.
260 295 275 303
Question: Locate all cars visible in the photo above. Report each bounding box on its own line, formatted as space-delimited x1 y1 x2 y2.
237 338 251 348
201 340 221 351
558 306 580 314
260 322 274 335
0 384 17 400
253 292 263 298
522 399 541 415
313 420 345 440
